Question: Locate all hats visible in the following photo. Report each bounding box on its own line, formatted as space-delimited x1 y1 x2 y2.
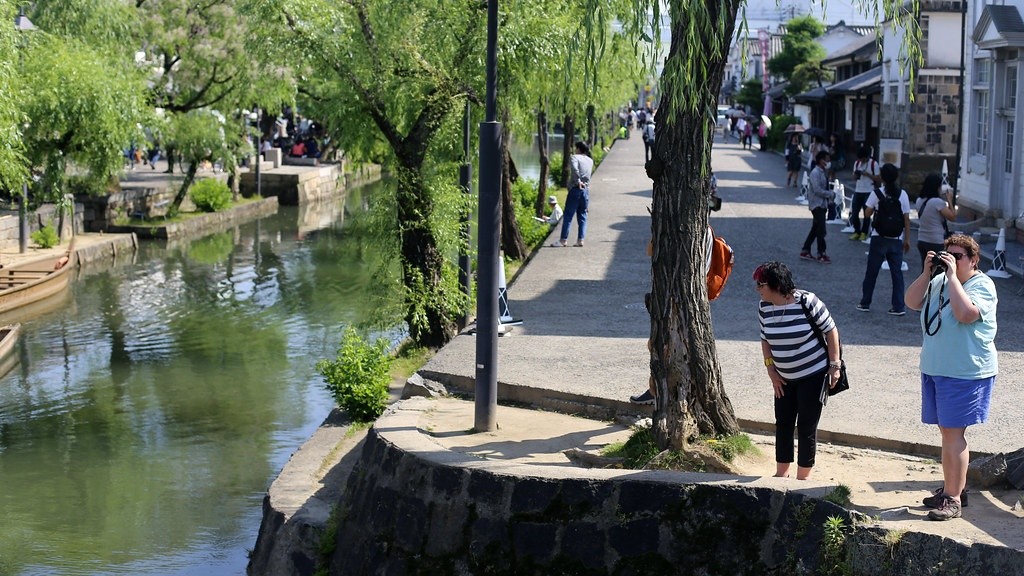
548 196 558 205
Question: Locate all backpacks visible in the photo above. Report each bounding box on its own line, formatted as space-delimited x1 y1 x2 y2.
871 187 905 238
706 224 735 302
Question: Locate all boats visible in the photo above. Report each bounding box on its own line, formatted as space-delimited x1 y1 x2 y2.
0 321 24 357
0 215 77 316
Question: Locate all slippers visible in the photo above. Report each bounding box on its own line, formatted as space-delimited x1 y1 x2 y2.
550 240 566 247
573 242 584 247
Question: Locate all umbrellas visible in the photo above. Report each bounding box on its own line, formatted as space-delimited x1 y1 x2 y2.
726 109 747 118
761 115 772 131
784 124 827 137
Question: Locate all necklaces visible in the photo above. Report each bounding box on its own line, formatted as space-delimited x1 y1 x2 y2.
773 298 788 323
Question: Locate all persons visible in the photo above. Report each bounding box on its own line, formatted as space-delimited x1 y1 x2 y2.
916 174 957 281
629 224 713 406
856 164 911 315
753 261 841 481
551 141 594 247
904 233 998 521
122 104 338 173
785 133 880 265
721 114 769 152
543 196 563 231
620 105 657 161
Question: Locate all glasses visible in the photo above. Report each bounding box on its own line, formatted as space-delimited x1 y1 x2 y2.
757 281 768 288
947 251 968 260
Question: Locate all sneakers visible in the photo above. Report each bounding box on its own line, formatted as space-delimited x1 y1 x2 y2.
857 305 870 312
923 487 968 508
927 493 962 521
887 308 906 315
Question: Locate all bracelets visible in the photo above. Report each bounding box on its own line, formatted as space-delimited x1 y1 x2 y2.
829 364 841 369
764 358 774 366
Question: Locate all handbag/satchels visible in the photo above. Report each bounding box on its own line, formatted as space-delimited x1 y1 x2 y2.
826 347 849 396
642 133 649 143
826 201 841 221
709 196 722 211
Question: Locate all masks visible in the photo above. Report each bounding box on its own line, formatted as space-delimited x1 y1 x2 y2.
822 161 831 169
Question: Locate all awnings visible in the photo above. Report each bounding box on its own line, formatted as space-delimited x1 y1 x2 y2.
793 65 883 106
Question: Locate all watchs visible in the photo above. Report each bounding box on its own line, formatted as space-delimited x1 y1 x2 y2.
830 361 842 367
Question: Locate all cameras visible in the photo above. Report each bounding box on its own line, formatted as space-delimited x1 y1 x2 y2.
932 252 949 268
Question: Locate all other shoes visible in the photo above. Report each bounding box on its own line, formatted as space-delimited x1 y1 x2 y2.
800 251 816 261
796 196 808 205
629 390 654 405
817 252 831 264
850 232 866 241
